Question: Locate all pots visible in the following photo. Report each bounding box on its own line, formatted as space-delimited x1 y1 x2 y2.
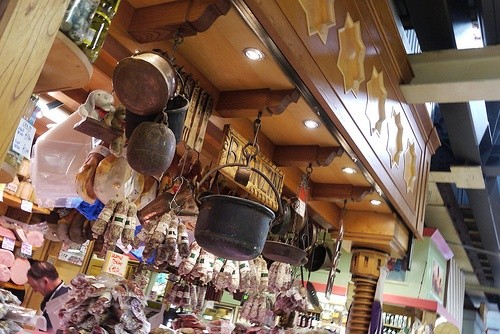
194 194 276 261
304 229 327 272
125 94 190 145
263 240 308 265
113 52 175 116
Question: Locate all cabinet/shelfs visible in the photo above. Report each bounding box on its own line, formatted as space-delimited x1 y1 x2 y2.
0 0 95 173
0 192 105 310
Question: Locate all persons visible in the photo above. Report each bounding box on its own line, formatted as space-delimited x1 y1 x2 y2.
26 262 72 333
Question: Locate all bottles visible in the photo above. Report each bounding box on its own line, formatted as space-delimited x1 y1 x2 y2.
59 0 101 45
78 0 121 64
